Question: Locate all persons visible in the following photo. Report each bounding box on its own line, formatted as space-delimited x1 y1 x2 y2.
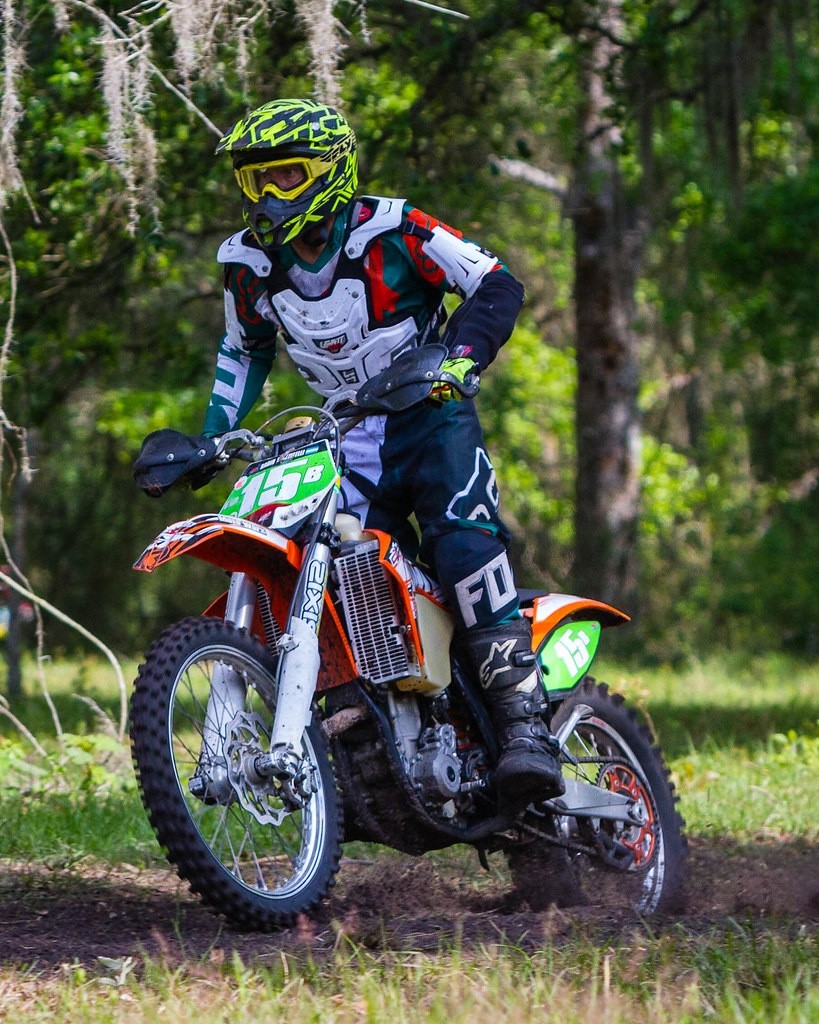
172 99 566 803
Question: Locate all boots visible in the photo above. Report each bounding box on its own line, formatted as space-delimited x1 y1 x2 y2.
466 620 567 800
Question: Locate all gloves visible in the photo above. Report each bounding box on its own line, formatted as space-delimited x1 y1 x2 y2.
428 356 480 402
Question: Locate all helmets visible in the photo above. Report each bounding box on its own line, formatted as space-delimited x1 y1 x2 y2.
213 98 357 249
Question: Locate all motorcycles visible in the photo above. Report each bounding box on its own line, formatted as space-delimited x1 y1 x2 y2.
127 343 686 934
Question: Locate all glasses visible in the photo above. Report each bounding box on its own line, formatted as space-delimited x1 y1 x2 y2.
235 158 318 202
